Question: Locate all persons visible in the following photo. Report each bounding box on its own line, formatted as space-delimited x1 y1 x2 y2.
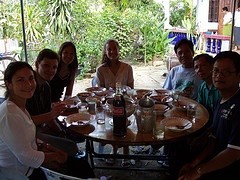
232 2 240 52
160 40 240 180
95 40 135 168
25 48 78 158
221 7 233 36
0 61 113 180
51 42 78 102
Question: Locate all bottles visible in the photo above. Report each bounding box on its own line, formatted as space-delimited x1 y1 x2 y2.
138 95 153 132
112 81 127 136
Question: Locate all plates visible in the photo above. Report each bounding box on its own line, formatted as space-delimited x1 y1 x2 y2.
65 114 95 126
161 116 192 131
151 95 173 103
86 87 106 93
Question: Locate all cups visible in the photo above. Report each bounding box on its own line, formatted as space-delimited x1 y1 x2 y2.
96 108 105 124
187 103 197 117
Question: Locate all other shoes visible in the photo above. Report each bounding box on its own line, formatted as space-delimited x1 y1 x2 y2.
106 151 119 164
158 155 170 168
121 159 131 168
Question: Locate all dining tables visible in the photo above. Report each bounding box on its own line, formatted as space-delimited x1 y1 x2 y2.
55 89 210 180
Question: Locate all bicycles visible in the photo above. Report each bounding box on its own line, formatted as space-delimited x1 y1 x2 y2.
0 39 50 90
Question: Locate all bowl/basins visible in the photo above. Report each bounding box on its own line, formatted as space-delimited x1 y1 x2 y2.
153 104 170 115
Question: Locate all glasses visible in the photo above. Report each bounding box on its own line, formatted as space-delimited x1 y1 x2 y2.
210 70 236 78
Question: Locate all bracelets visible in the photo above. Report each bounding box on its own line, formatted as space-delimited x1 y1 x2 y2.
193 167 201 176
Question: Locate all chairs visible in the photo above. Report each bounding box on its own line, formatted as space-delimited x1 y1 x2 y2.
91 77 104 154
